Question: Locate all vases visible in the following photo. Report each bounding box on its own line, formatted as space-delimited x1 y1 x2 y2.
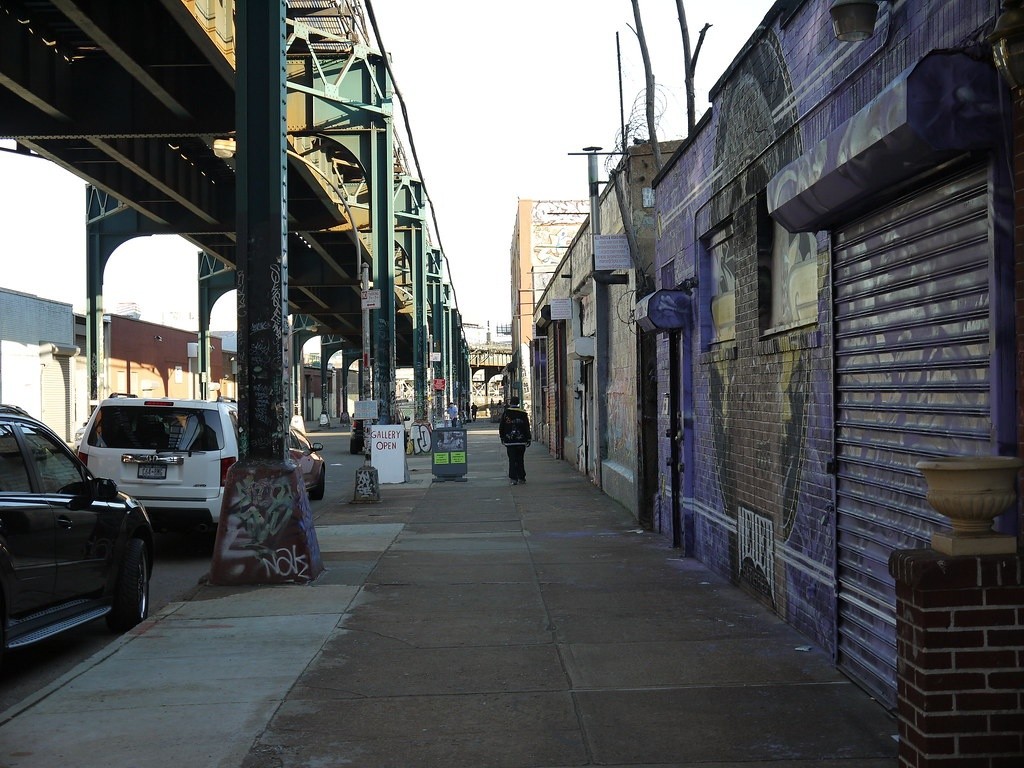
916 455 1019 559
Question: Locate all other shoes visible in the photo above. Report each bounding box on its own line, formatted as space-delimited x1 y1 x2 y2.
519 479 525 484
511 479 518 485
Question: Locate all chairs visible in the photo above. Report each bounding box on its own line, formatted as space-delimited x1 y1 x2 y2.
132 412 171 449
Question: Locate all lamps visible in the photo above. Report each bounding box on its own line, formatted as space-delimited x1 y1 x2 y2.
829 0 879 42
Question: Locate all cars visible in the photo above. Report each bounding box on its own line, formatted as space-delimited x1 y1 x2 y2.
290 425 326 500
349 410 410 454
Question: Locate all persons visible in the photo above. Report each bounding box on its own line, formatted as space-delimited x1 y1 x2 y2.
447 403 458 427
499 396 532 486
178 412 219 450
489 398 510 414
459 401 478 423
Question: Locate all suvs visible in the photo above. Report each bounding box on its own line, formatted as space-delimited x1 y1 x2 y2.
0 403 155 653
77 392 238 556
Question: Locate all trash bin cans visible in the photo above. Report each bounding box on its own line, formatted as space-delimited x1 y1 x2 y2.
430 428 468 484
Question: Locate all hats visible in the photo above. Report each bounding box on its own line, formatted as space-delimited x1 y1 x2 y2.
510 397 519 404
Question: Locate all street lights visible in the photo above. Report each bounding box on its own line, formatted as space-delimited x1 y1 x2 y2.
213 135 373 404
